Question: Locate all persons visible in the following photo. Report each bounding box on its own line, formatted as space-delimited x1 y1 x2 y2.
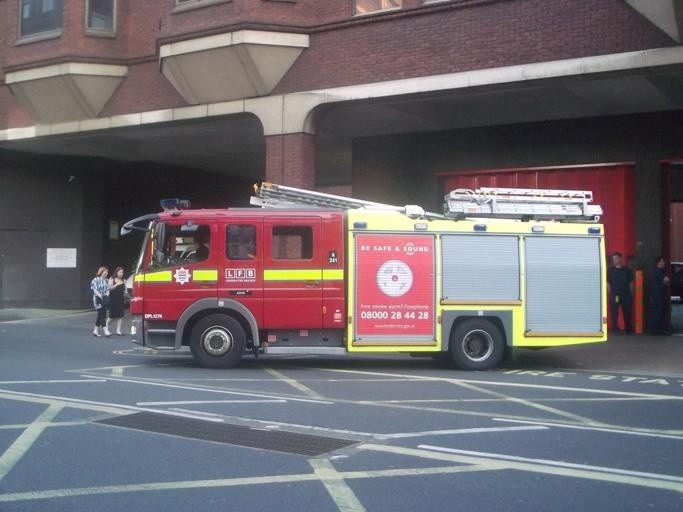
606 252 635 335
89 266 113 337
648 255 671 337
106 267 129 336
188 231 208 261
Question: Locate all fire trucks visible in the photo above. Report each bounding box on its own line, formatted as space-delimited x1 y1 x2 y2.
120 181 609 371
